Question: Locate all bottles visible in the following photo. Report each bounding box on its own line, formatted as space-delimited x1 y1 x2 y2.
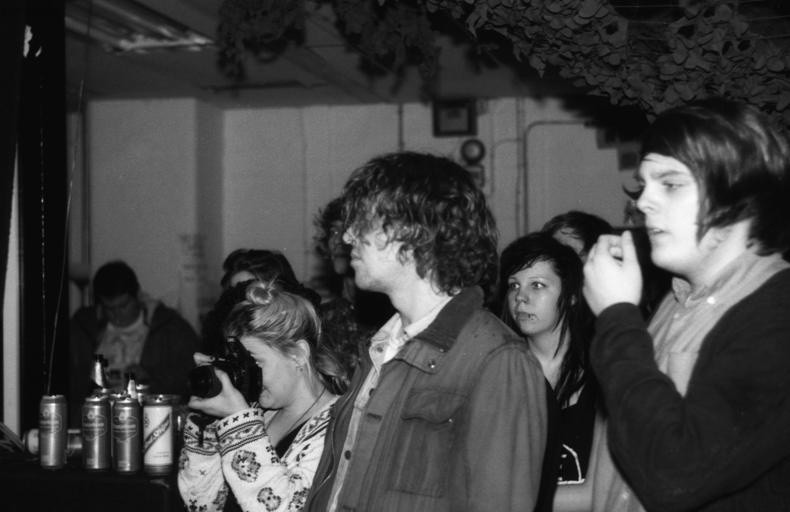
90 352 139 401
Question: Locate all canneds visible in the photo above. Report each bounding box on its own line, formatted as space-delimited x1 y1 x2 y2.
22 385 185 477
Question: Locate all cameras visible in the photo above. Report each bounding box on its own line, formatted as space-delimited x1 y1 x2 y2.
187 337 262 404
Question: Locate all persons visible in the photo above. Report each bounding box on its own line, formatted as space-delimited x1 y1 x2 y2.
310 195 398 396
581 98 790 510
175 278 353 512
299 148 549 512
541 208 612 255
498 231 603 512
61 258 202 416
220 249 297 292
